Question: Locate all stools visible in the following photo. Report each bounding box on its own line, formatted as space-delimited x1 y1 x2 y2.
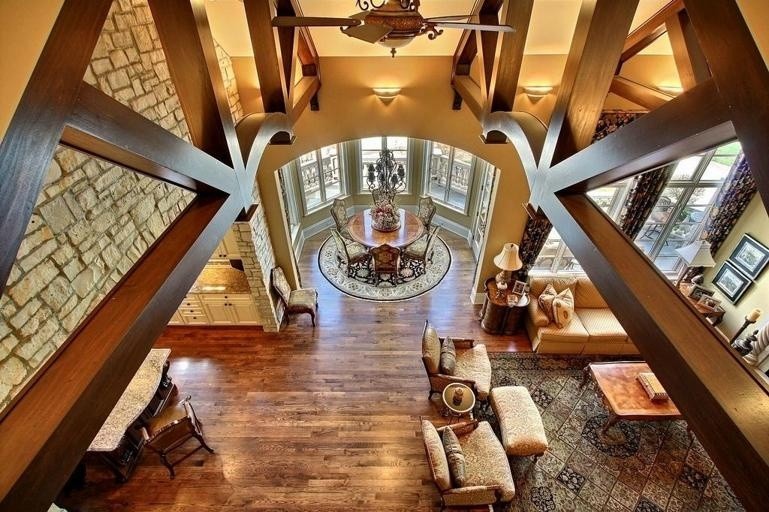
485 385 546 464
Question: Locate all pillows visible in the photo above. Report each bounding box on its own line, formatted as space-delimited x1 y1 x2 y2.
438 335 454 375
537 284 574 328
441 424 464 484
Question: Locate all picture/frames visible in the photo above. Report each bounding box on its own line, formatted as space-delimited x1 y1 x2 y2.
687 233 769 312
511 280 526 297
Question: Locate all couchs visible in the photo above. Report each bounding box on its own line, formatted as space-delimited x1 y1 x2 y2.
526 275 640 354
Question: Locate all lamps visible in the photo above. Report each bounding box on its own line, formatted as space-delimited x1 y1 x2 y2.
364 137 407 203
374 88 402 107
673 240 716 288
522 84 551 105
492 243 523 288
364 1 423 62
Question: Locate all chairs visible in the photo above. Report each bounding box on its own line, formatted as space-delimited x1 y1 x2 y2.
136 395 214 480
270 267 318 328
418 416 515 511
419 319 491 409
329 184 441 288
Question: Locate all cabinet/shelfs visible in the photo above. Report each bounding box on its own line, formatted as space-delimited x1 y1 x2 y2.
166 295 260 327
208 228 240 262
479 278 529 336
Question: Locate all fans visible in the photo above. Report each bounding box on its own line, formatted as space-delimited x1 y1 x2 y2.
273 1 517 45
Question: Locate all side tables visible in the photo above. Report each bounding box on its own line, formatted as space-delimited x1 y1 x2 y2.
441 382 476 425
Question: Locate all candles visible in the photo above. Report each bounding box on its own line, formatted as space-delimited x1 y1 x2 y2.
747 309 761 322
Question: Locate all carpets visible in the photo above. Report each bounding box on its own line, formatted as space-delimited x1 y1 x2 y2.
464 351 742 511
318 225 451 303
